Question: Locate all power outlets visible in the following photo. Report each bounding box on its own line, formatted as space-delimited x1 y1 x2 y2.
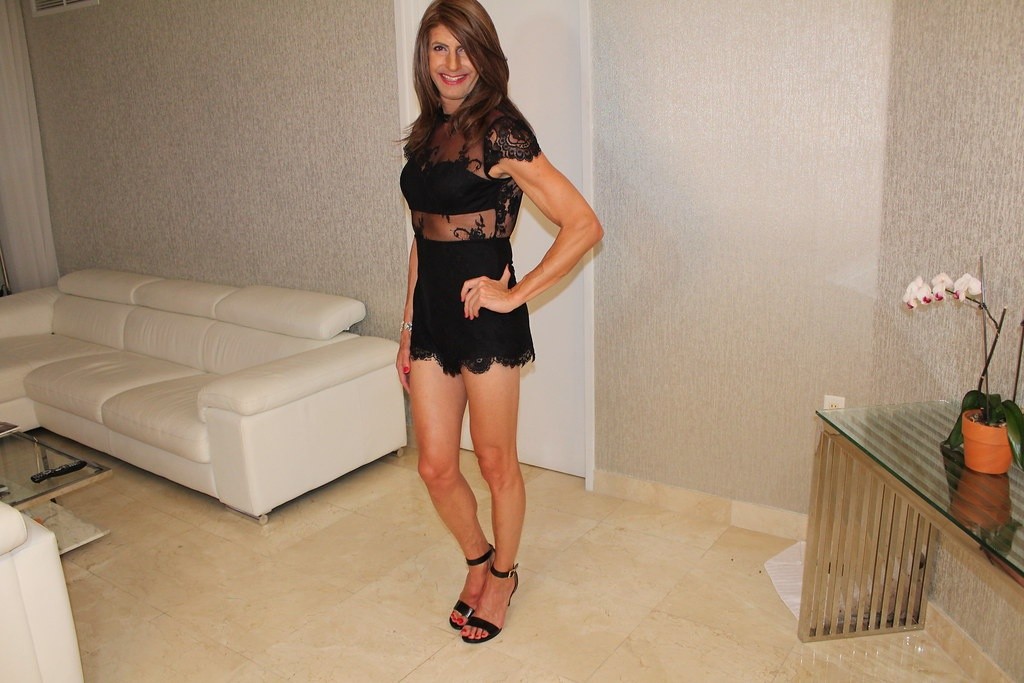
823 394 847 410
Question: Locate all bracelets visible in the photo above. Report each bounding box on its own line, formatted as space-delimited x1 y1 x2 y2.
400 320 412 332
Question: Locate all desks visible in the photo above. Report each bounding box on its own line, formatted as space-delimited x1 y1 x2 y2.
796 403 1024 646
0 421 114 556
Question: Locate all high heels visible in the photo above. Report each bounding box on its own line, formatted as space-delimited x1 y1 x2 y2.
462 563 519 643
449 543 496 630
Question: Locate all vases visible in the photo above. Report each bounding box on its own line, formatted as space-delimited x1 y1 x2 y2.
959 409 1015 475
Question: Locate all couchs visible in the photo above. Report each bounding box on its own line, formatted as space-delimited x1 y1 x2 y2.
0 499 85 683
0 269 408 527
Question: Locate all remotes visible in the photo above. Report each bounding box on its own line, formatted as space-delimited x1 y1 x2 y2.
30 461 87 483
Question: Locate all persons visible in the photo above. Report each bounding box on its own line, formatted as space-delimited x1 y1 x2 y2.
396 0 604 644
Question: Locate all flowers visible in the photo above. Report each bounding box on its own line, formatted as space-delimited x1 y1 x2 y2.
901 268 1024 472
